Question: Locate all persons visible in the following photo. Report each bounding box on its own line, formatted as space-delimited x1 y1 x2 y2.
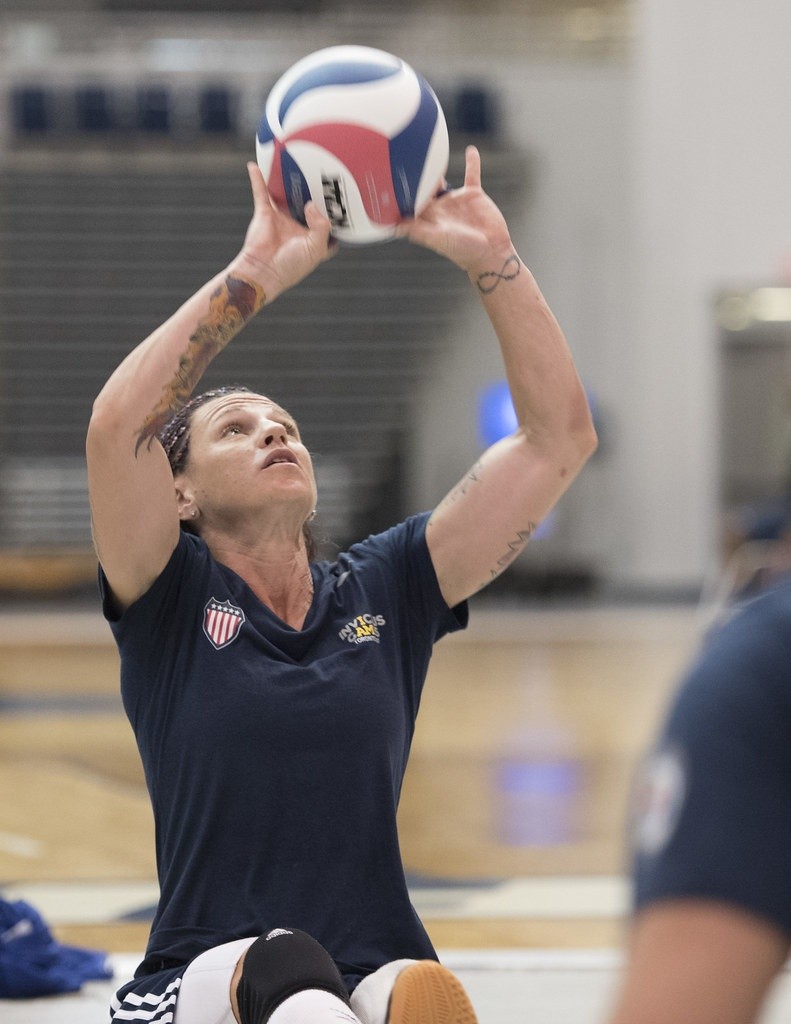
84 140 600 1024
609 561 791 1024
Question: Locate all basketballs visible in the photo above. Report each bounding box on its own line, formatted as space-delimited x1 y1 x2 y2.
254 42 452 245
